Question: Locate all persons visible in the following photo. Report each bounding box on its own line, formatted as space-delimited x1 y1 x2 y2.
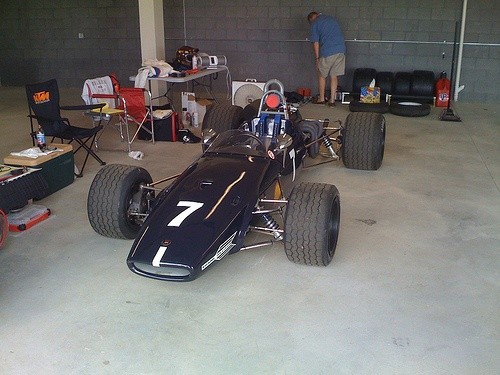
308 11 346 108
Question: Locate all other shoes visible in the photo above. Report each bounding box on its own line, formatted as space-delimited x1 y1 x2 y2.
329 100 335 108
312 100 325 104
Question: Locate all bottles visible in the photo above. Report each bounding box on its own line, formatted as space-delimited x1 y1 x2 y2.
182 108 188 124
335 87 342 101
191 55 198 69
193 112 199 128
435 71 451 107
35 125 47 152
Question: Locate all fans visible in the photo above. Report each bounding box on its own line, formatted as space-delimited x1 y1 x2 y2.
232 79 266 109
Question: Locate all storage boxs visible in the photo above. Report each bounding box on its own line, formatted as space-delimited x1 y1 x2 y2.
360 87 381 103
174 91 196 128
0 144 75 214
137 103 178 140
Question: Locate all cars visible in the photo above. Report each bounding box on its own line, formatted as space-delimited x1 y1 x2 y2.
87 79 386 282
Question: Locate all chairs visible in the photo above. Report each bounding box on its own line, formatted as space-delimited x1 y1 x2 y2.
25 79 106 178
81 76 154 152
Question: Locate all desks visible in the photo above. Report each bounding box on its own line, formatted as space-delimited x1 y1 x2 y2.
130 67 232 104
